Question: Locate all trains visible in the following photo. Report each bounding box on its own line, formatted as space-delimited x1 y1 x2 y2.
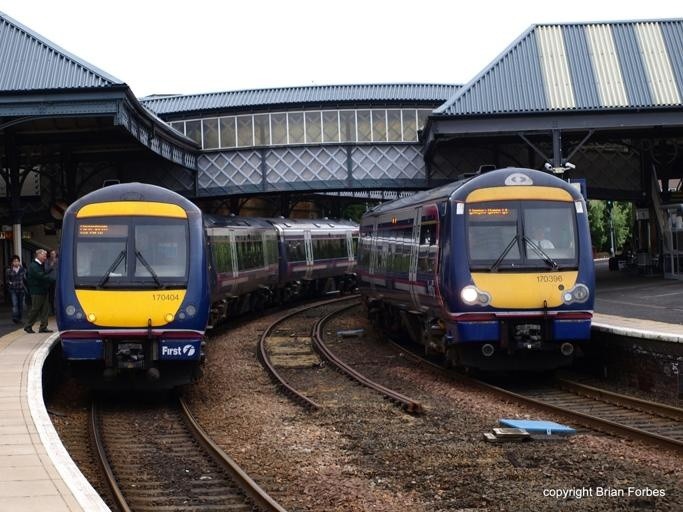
53 177 361 396
360 162 600 386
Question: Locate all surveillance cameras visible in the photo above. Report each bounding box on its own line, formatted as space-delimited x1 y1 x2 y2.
543 161 553 171
565 161 576 170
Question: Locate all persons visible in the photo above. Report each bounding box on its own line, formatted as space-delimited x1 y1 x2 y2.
526 227 555 250
22 249 58 333
43 250 58 317
3 255 27 323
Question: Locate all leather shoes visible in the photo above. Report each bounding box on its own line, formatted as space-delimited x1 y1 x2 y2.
24 327 35 333
39 328 51 333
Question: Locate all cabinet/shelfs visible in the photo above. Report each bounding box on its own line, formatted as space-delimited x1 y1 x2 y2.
659 203 683 280
